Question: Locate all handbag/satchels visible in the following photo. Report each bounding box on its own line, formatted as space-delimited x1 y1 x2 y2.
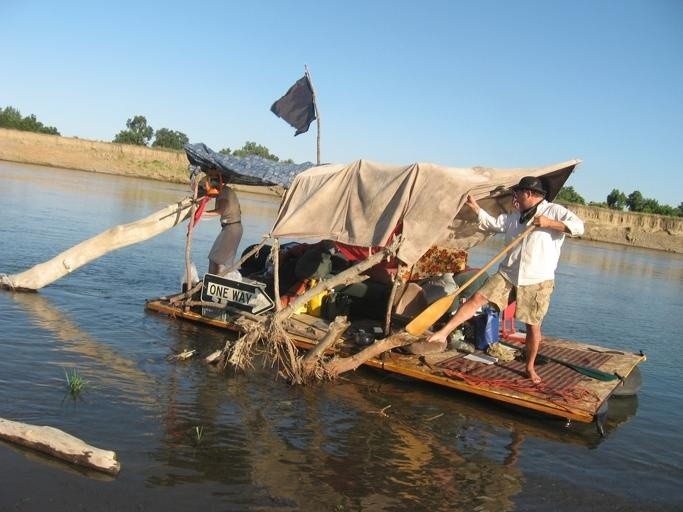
240 239 498 355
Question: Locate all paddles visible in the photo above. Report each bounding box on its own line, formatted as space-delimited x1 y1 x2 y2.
406 225 535 338
500 340 616 381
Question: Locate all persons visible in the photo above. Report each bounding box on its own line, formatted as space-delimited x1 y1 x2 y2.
423 176 585 385
200 176 244 276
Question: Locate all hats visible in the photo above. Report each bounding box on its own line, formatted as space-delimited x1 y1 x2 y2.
513 176 547 194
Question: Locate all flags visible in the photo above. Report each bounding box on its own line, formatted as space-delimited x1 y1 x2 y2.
269 75 317 137
